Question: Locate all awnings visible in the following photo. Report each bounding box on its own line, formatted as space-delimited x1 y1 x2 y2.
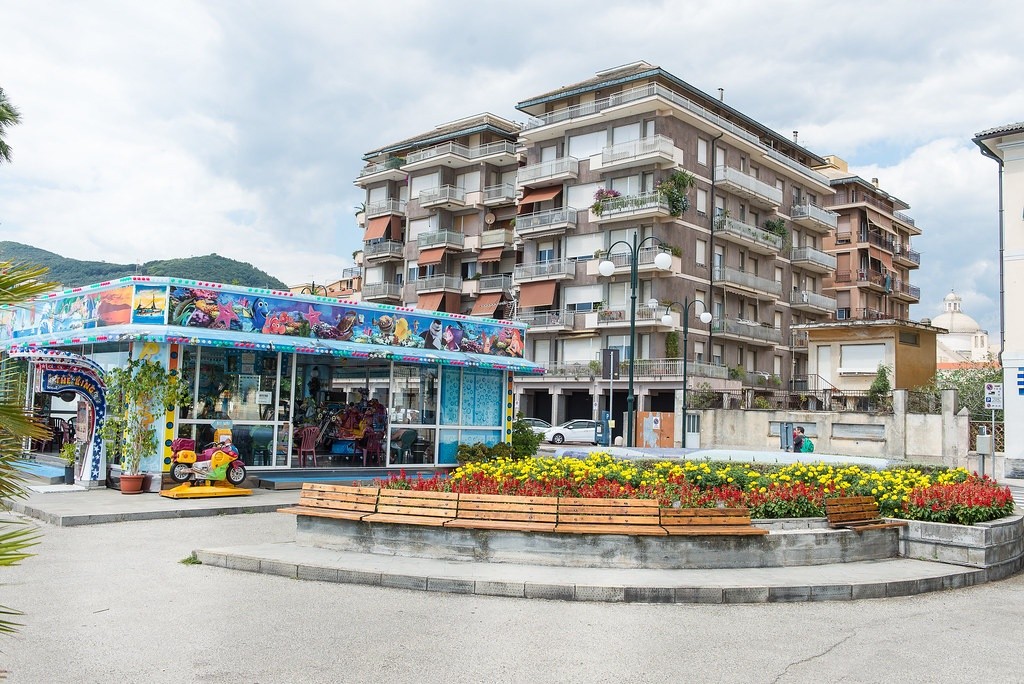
362 184 558 318
868 217 900 275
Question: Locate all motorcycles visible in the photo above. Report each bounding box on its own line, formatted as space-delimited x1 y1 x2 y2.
170 437 247 484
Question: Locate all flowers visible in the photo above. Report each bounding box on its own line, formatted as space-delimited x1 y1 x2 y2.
653 178 665 191
607 310 623 317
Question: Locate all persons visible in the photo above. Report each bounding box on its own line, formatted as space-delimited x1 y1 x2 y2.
792 427 808 453
380 418 410 464
793 428 798 440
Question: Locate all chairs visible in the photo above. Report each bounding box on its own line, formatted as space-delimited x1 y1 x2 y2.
68 417 77 445
284 426 320 468
391 430 416 464
352 428 385 467
252 426 272 465
48 417 66 436
34 416 61 454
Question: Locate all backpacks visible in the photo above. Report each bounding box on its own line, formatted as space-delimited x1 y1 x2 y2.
799 435 814 453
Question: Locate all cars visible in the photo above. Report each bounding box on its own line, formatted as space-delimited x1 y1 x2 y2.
513 418 553 440
541 419 604 445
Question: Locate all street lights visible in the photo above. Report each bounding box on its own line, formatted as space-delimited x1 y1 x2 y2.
598 230 673 448
660 295 713 450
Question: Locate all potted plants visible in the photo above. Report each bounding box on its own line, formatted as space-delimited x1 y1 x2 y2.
654 368 667 374
768 374 782 387
96 356 196 495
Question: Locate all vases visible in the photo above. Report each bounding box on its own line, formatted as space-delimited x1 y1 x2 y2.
593 188 621 203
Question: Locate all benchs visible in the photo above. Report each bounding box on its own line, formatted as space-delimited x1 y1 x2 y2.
362 489 458 527
555 497 668 536
825 495 909 532
659 508 769 536
277 482 379 521
444 494 558 533
